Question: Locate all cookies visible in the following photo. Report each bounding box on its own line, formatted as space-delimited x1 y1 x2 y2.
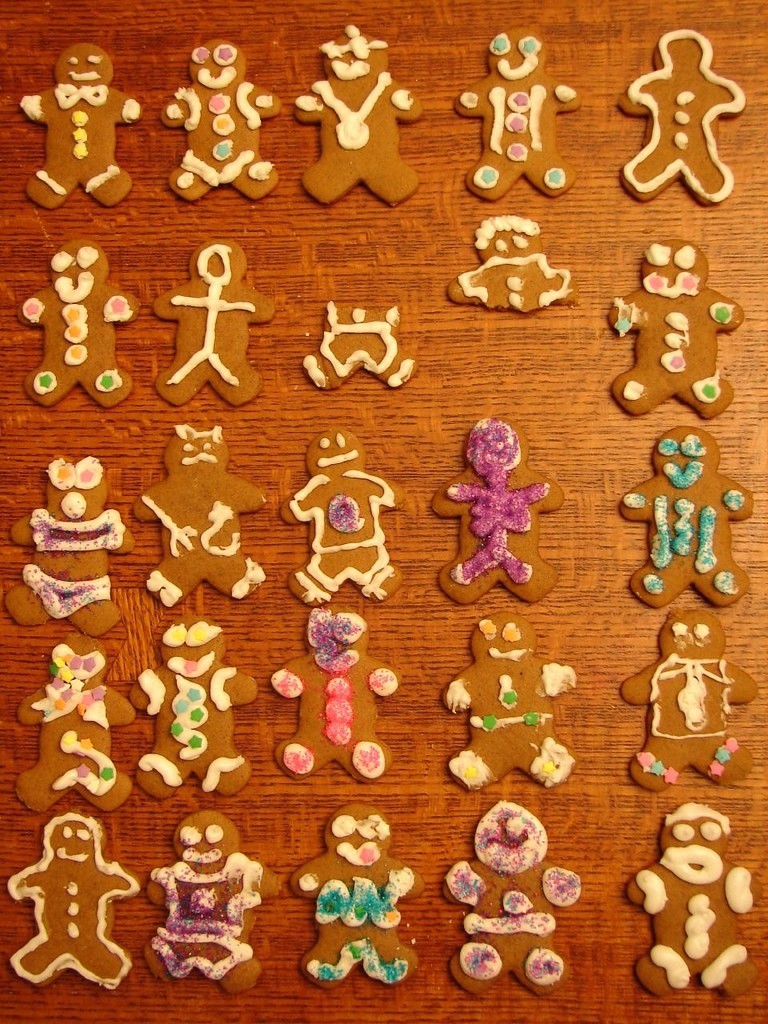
4 25 761 996
